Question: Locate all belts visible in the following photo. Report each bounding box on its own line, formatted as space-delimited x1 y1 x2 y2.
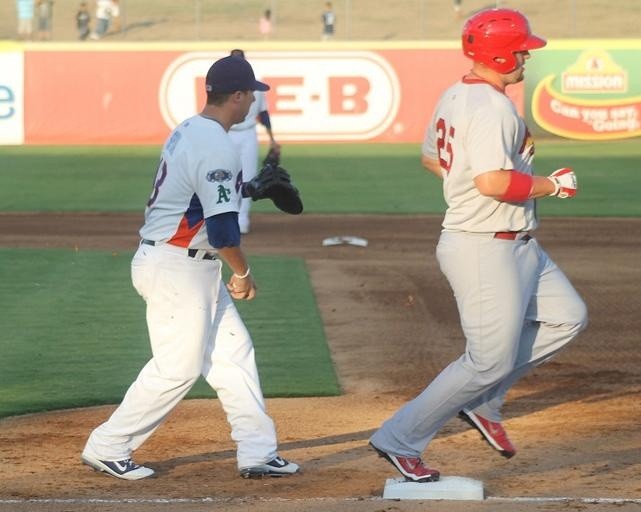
143 239 215 260
494 232 529 240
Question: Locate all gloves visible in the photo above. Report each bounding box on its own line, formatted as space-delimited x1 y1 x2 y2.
546 167 577 200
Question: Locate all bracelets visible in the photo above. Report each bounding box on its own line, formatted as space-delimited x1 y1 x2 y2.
233 265 252 282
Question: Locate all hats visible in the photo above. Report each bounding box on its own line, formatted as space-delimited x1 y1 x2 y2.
205 55 270 92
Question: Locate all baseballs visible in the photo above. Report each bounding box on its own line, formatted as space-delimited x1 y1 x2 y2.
232 283 250 296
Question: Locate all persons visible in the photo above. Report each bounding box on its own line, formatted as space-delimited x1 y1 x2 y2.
79 55 302 484
16 0 126 46
257 7 274 41
318 2 338 42
368 12 590 480
452 0 470 25
224 46 283 235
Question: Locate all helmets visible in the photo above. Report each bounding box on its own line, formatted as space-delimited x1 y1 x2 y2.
461 7 547 74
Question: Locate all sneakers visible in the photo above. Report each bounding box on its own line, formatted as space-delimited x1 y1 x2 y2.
239 456 300 479
456 407 516 459
369 441 439 483
81 452 155 481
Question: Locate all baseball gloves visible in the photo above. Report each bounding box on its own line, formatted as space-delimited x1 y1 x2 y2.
262 143 281 166
242 167 303 214
